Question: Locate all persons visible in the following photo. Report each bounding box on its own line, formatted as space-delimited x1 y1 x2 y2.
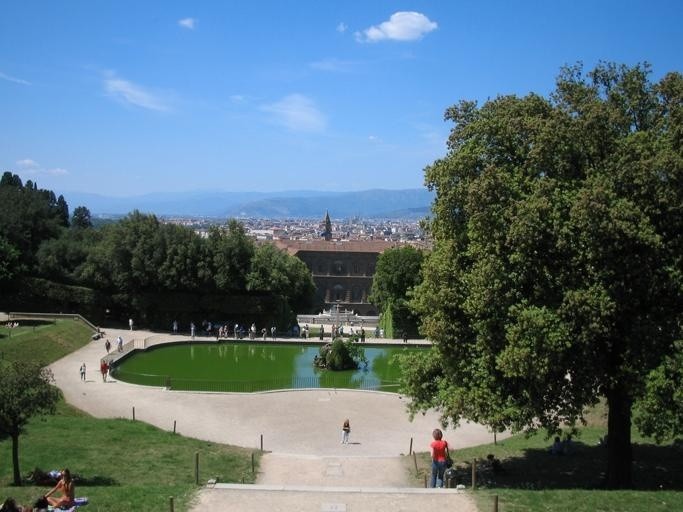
79 363 87 380
100 360 110 382
104 340 111 352
170 321 277 341
401 331 408 343
0 465 75 512
299 323 386 344
116 337 125 352
430 428 450 488
342 418 351 444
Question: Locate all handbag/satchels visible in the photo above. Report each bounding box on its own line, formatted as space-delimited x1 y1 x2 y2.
447 456 453 468
